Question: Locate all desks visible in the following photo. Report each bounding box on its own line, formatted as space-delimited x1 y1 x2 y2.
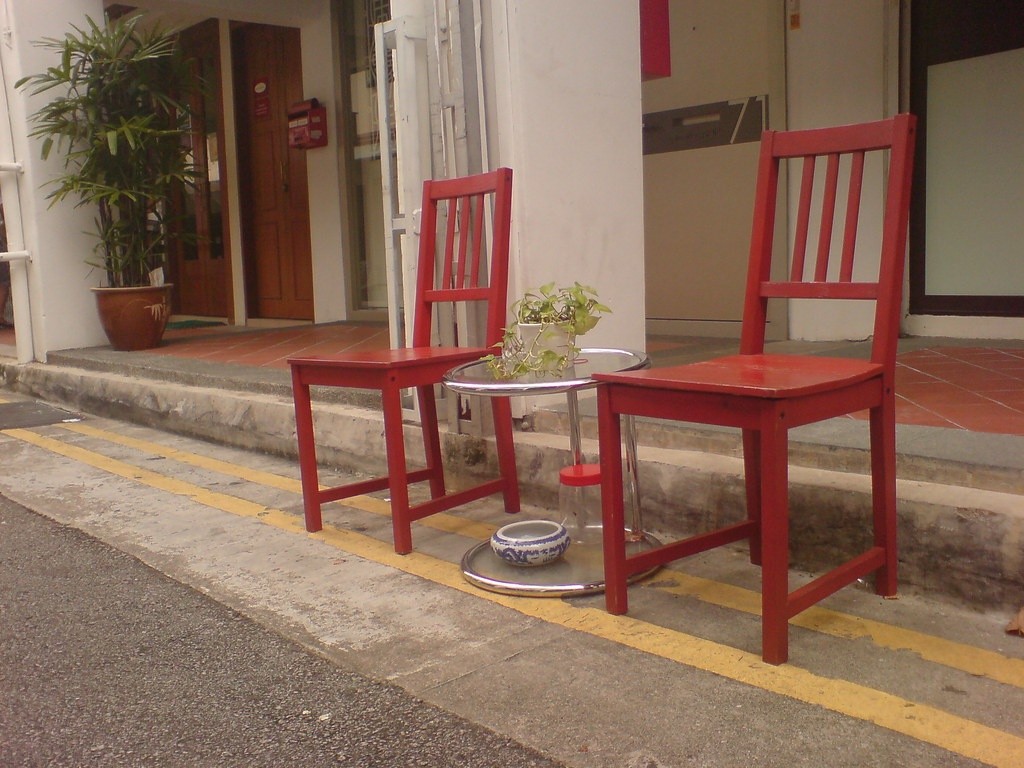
440 347 665 599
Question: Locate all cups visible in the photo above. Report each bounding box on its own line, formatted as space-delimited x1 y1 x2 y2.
559 463 603 544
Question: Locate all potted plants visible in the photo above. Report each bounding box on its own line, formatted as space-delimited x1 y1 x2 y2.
13 10 217 352
479 280 612 381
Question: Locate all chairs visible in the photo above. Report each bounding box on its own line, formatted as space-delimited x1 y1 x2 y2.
589 112 918 665
284 166 522 557
